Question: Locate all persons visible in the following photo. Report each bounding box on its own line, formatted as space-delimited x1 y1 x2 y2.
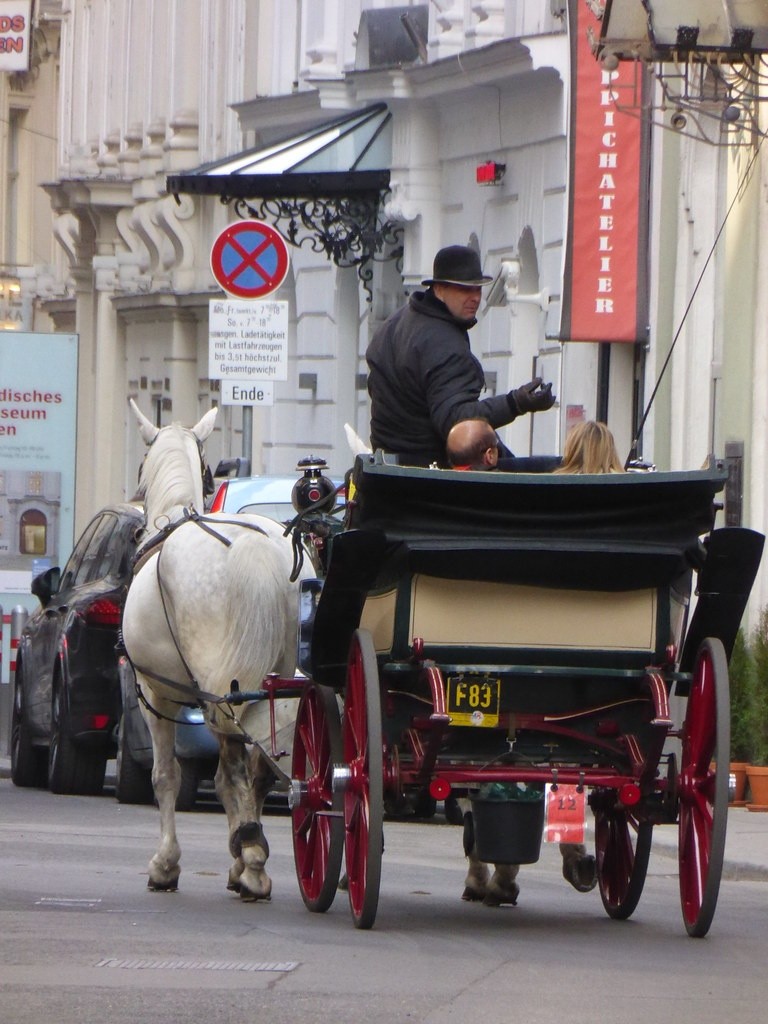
366 245 627 474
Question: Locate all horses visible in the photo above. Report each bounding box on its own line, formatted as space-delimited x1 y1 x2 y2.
120 394 318 904
443 772 604 912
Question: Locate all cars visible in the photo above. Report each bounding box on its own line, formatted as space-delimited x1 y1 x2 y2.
112 472 346 808
7 501 153 806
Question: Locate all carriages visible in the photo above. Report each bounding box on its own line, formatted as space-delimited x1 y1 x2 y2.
113 395 768 939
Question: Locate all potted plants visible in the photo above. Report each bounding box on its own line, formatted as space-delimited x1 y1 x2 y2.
708 603 768 805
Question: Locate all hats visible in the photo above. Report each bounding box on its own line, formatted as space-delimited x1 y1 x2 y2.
420 245 494 287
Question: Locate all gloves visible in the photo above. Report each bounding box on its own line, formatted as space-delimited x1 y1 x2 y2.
509 377 557 415
